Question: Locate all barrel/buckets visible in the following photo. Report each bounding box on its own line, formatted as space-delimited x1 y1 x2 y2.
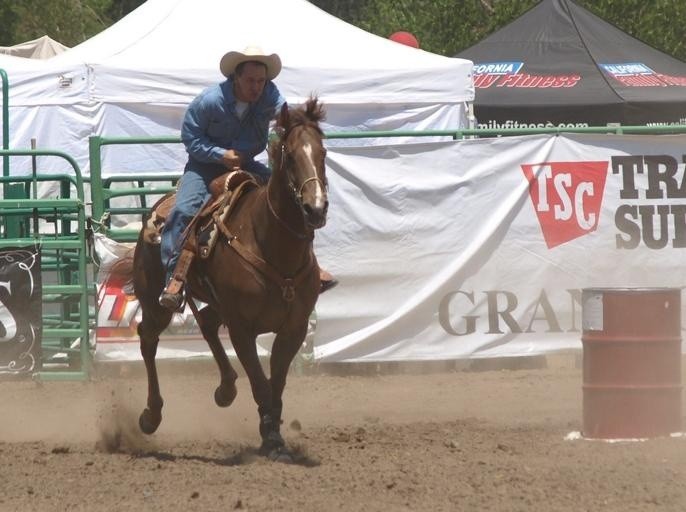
581 286 682 436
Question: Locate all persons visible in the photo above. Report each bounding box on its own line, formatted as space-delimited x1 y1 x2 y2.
158 50 338 314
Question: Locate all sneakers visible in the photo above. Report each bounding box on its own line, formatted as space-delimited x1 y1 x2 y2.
161 292 182 311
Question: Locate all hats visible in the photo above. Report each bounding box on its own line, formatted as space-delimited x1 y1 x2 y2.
219 47 281 81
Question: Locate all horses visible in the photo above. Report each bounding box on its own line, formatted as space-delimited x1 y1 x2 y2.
114 91 330 465
